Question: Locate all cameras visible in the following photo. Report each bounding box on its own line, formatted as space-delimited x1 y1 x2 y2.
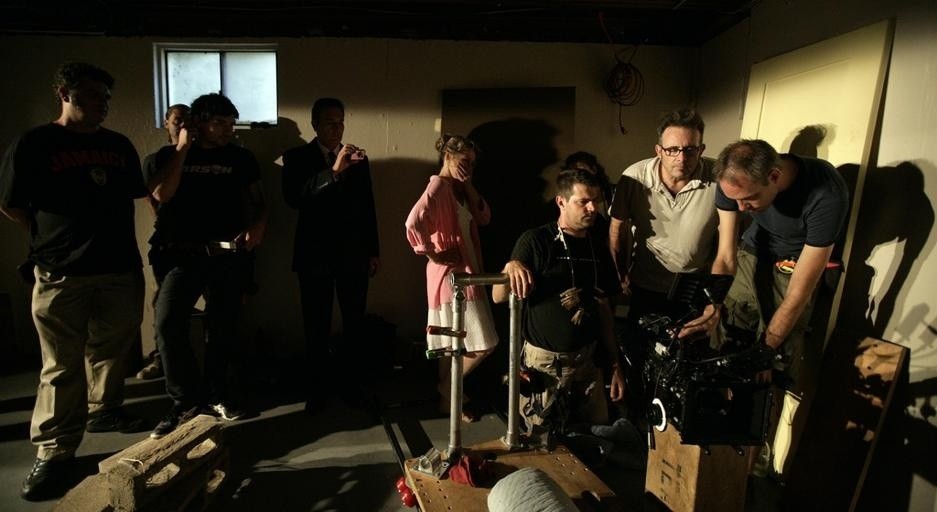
350 149 365 160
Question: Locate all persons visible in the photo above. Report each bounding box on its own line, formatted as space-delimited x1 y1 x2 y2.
136 94 269 435
283 96 381 404
3 65 149 499
490 108 848 488
404 133 500 423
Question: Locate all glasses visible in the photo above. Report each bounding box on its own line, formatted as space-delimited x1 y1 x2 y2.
660 143 702 155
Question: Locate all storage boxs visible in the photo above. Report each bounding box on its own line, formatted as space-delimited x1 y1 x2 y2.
642 413 756 511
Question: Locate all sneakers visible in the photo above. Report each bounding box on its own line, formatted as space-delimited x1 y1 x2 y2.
86 410 150 433
136 364 161 379
21 459 65 499
151 401 198 439
208 396 244 421
